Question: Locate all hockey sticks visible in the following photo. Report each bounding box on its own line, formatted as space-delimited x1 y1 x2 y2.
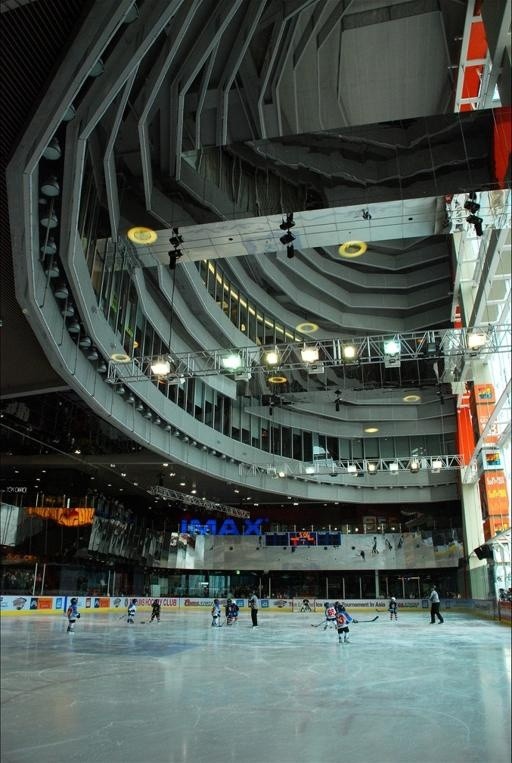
358 617 378 623
140 615 152 624
218 613 222 627
311 620 327 628
375 610 389 613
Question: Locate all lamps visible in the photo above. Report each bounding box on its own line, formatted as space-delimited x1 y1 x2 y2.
87 347 98 360
126 390 134 406
42 208 57 229
43 237 56 255
80 335 90 348
105 373 114 385
45 261 59 278
54 282 70 301
122 4 139 26
143 405 151 419
68 318 80 334
154 413 160 426
62 303 73 316
135 398 142 412
116 384 124 394
62 104 76 122
97 361 106 372
44 136 62 161
89 59 105 77
41 176 59 195
152 357 170 375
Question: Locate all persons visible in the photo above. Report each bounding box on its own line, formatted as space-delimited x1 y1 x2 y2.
428 585 444 625
227 590 235 598
66 597 80 634
210 599 220 626
149 599 161 625
301 599 311 612
127 599 139 624
388 596 398 621
323 601 338 629
225 599 240 625
248 590 259 628
335 604 358 643
173 583 184 597
